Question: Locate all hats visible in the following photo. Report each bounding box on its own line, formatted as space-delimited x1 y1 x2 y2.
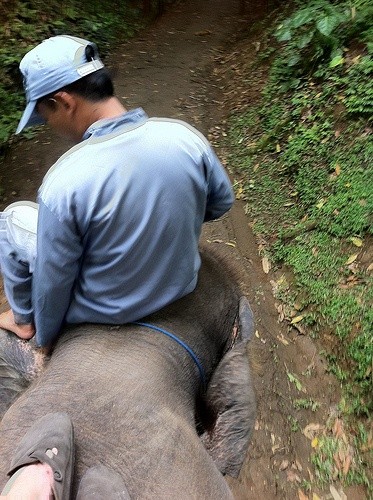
14 34 105 135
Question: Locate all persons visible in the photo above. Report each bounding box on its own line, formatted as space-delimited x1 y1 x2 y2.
0 413 132 500
0 35 236 350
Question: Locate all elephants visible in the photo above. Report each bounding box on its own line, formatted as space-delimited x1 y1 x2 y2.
0 243 260 500
